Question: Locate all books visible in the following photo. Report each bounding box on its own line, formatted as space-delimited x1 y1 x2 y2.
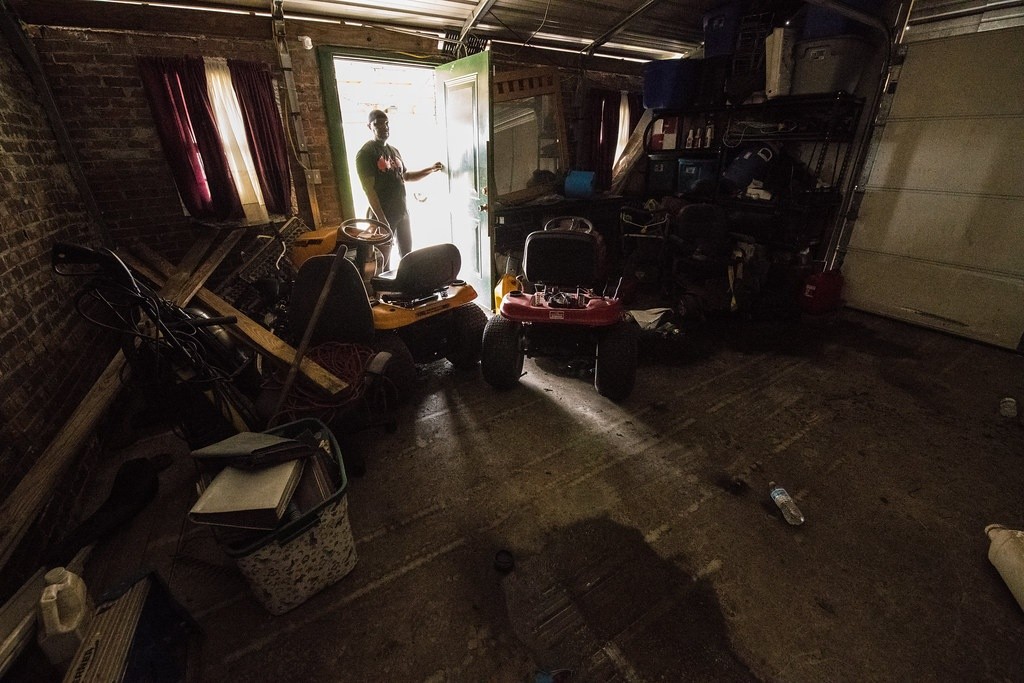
188 432 334 532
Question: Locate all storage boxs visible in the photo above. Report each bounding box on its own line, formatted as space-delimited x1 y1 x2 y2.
193 417 357 617
793 36 879 99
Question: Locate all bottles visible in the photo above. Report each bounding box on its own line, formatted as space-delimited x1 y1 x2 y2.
769 481 805 526
685 127 712 148
1000 398 1016 418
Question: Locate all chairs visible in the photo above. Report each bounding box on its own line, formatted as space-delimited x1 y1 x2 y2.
522 229 599 288
292 254 375 344
370 244 461 297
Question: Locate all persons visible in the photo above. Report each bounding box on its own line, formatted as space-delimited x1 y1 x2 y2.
356 110 444 273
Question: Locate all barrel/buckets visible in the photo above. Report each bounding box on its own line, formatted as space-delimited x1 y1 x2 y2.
701 3 741 59
678 157 715 193
647 154 678 198
640 60 686 109
718 141 784 199
34 567 93 674
798 267 844 316
565 170 596 198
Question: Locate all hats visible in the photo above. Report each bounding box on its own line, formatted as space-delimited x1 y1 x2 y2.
368 110 388 123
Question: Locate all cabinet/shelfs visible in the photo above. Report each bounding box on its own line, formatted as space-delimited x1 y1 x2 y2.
496 193 623 281
649 90 868 256
